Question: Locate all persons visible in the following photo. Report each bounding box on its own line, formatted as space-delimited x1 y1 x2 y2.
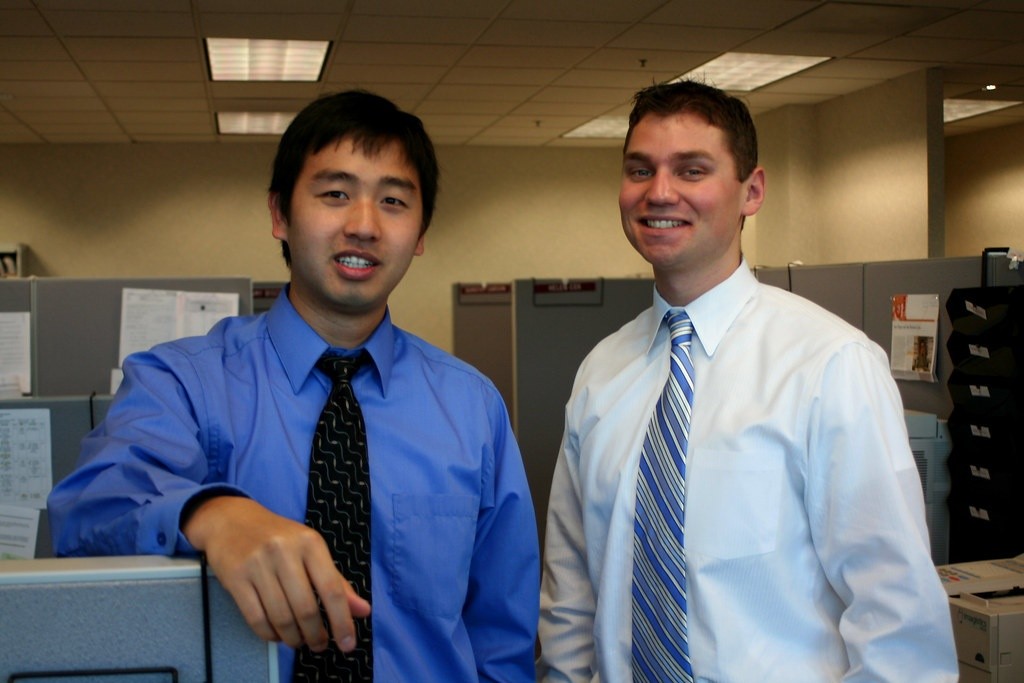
543 81 958 683
47 91 540 683
910 336 928 369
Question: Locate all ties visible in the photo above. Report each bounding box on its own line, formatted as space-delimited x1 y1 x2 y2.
630 310 694 683
288 350 373 683
